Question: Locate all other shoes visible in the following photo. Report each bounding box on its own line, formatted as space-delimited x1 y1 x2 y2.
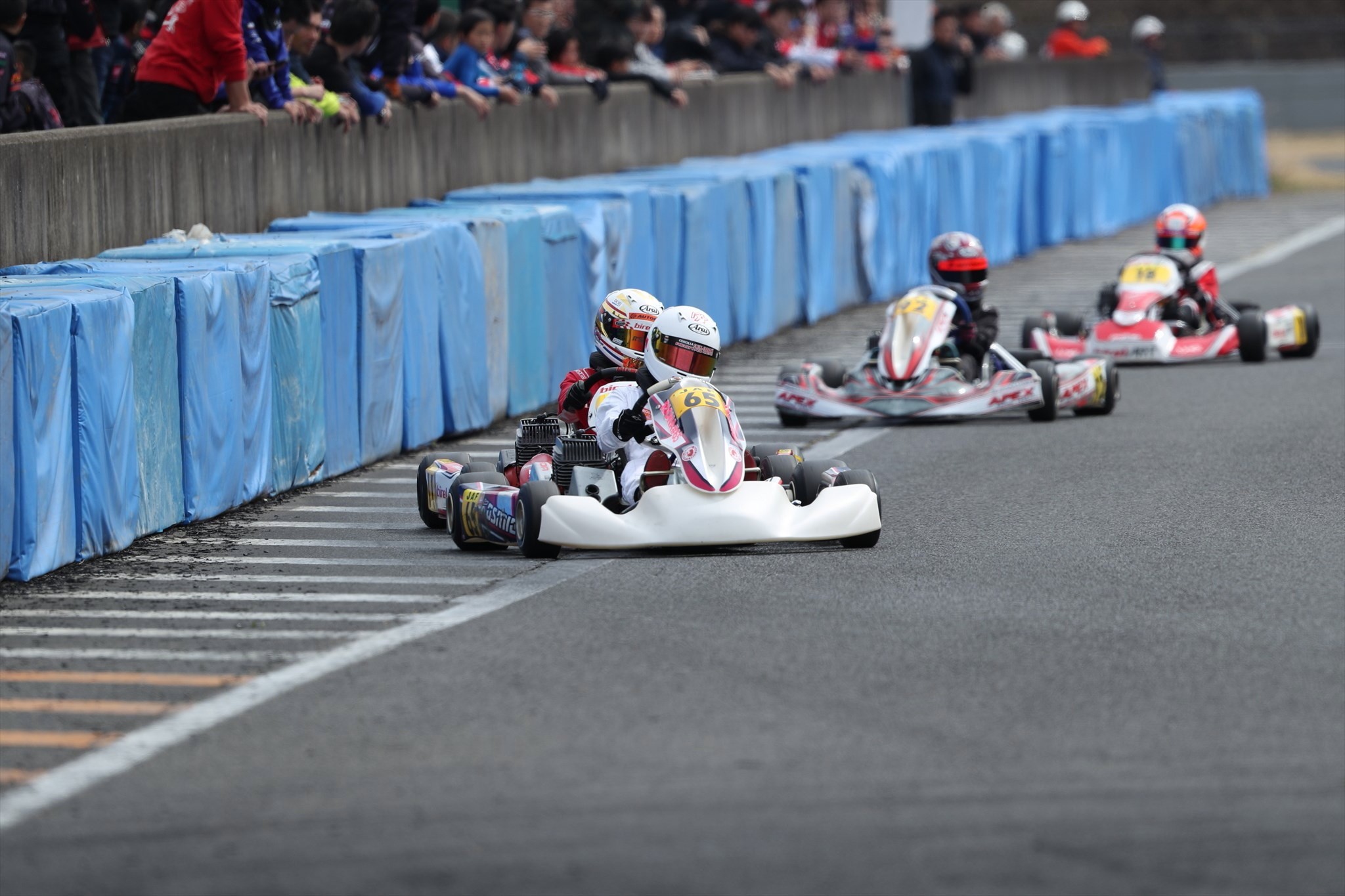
744 450 758 481
644 450 670 488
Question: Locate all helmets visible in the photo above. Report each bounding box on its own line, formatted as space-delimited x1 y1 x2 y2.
997 30 1027 61
644 306 720 386
1130 15 1167 40
594 289 664 369
928 231 989 302
1155 202 1208 267
1056 0 1089 20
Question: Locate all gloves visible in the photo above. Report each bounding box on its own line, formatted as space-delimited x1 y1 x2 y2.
616 409 647 441
567 380 591 410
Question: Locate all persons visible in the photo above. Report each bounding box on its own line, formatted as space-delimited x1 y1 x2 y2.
557 288 662 434
0 0 1165 140
868 231 998 382
1095 203 1219 337
595 306 758 506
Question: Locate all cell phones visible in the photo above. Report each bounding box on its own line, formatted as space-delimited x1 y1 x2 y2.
255 60 289 75
